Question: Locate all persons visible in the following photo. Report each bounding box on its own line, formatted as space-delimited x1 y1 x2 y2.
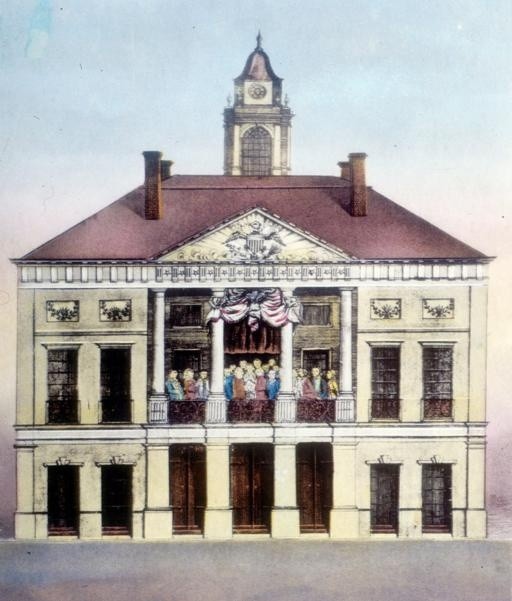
223 357 280 399
325 368 340 400
195 368 210 399
292 366 302 399
297 367 318 398
182 367 197 400
311 366 328 398
165 368 185 400
